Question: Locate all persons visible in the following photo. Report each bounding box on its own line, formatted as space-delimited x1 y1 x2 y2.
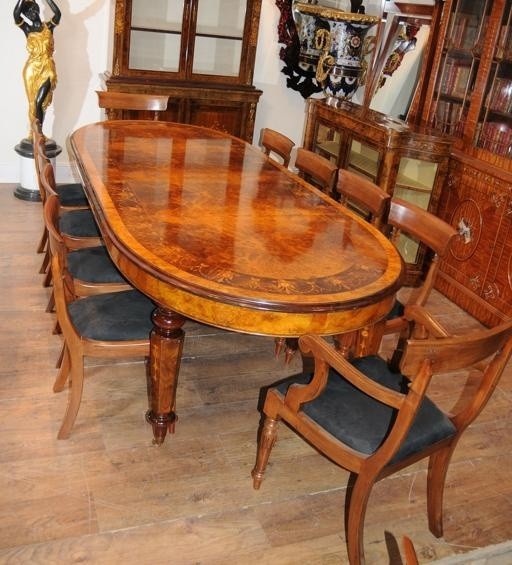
12 0 62 140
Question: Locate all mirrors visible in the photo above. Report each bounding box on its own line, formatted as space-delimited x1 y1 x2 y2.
275 1 366 97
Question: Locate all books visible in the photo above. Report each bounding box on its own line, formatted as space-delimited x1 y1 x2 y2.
426 10 512 161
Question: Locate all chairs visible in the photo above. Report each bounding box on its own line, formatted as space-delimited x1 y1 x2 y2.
40 165 104 310
251 314 510 565
328 169 391 235
96 89 170 121
292 146 336 201
42 195 134 368
36 129 91 273
280 197 460 371
32 136 104 286
257 126 295 172
40 231 151 442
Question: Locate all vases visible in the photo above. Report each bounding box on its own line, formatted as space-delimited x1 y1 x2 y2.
310 6 380 110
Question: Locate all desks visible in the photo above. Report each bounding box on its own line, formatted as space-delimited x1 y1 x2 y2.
69 119 405 444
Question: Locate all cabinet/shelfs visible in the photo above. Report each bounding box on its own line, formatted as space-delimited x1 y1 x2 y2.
299 98 449 287
421 0 511 332
